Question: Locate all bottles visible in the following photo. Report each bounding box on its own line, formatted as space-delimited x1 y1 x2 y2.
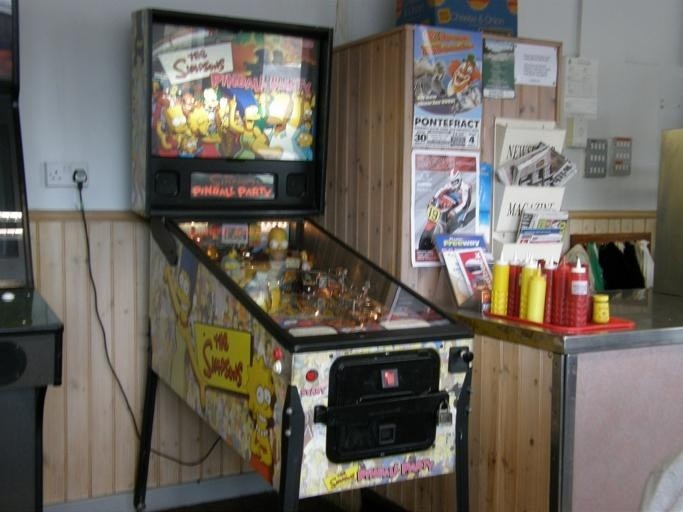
189 227 378 324
491 256 610 324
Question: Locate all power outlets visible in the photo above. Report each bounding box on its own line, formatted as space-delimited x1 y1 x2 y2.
44 161 93 188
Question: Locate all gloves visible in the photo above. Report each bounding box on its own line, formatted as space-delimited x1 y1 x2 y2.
446 211 455 219
429 196 437 208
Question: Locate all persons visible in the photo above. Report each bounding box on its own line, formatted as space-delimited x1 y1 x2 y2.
432 167 473 230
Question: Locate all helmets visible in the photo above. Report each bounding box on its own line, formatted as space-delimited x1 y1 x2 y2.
448 168 461 191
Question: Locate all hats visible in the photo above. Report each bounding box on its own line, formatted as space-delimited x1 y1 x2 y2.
462 55 476 66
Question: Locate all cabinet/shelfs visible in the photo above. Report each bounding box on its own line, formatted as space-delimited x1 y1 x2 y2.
419 305 682 510
326 23 565 511
